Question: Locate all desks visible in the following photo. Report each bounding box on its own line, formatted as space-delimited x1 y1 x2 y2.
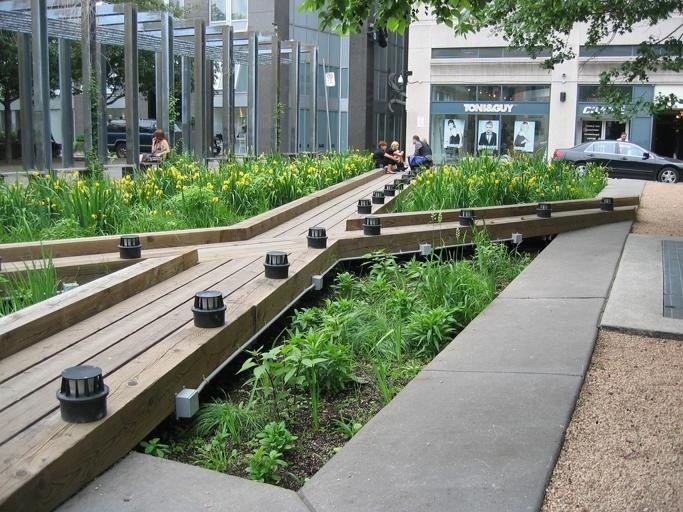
396 69 404 153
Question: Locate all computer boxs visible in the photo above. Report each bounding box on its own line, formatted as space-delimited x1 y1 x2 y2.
398 75 404 86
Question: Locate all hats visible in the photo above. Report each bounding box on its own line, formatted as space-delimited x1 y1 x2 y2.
108 118 221 158
553 140 683 183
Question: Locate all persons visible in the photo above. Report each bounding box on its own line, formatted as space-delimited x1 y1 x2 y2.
373 135 432 173
617 132 627 141
448 120 461 144
514 122 530 147
214 139 222 154
479 122 497 147
151 128 170 163
237 126 246 138
